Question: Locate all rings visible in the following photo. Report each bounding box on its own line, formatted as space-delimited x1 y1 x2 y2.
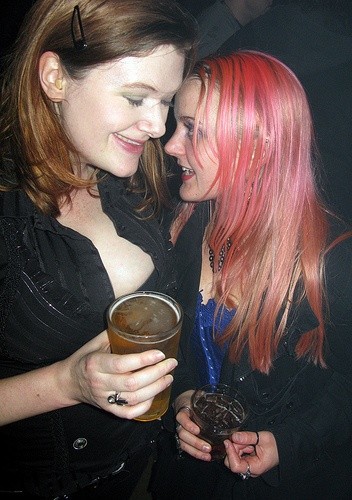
252 431 260 447
108 391 129 408
174 435 181 450
177 406 193 418
240 457 251 481
176 422 183 435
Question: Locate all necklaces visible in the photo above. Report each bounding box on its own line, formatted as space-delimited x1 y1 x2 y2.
206 225 232 273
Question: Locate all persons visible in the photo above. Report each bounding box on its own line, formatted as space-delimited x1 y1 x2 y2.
1 0 203 499
161 48 352 500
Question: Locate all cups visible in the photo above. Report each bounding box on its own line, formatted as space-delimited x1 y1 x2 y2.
107 290 184 422
189 382 251 462
